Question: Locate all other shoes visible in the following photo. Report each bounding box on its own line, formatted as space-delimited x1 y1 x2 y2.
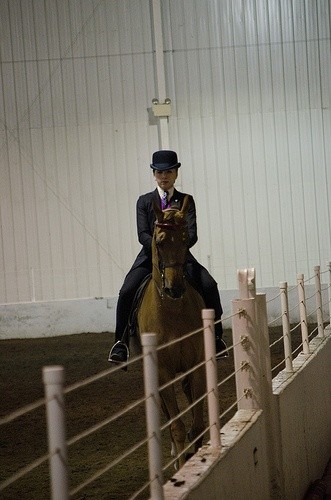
214 334 228 358
108 344 129 371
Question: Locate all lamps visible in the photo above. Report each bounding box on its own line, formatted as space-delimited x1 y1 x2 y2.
152 97 171 117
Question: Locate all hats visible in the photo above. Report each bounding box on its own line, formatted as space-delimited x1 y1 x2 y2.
150 150 181 170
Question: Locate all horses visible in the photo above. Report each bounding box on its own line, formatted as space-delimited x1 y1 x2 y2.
126 193 208 471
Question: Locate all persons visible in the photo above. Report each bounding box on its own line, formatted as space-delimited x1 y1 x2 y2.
111 149 227 362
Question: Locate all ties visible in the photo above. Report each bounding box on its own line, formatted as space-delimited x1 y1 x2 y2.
161 190 169 210
